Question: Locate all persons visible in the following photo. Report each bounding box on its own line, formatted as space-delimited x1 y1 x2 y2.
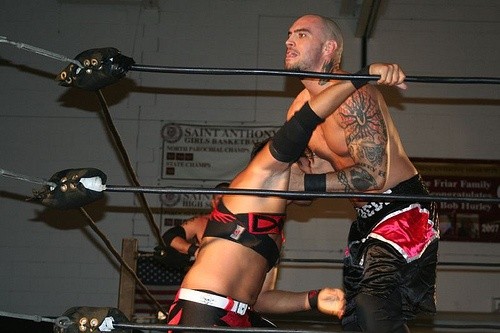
163 182 231 262
165 62 407 333
281 15 441 333
